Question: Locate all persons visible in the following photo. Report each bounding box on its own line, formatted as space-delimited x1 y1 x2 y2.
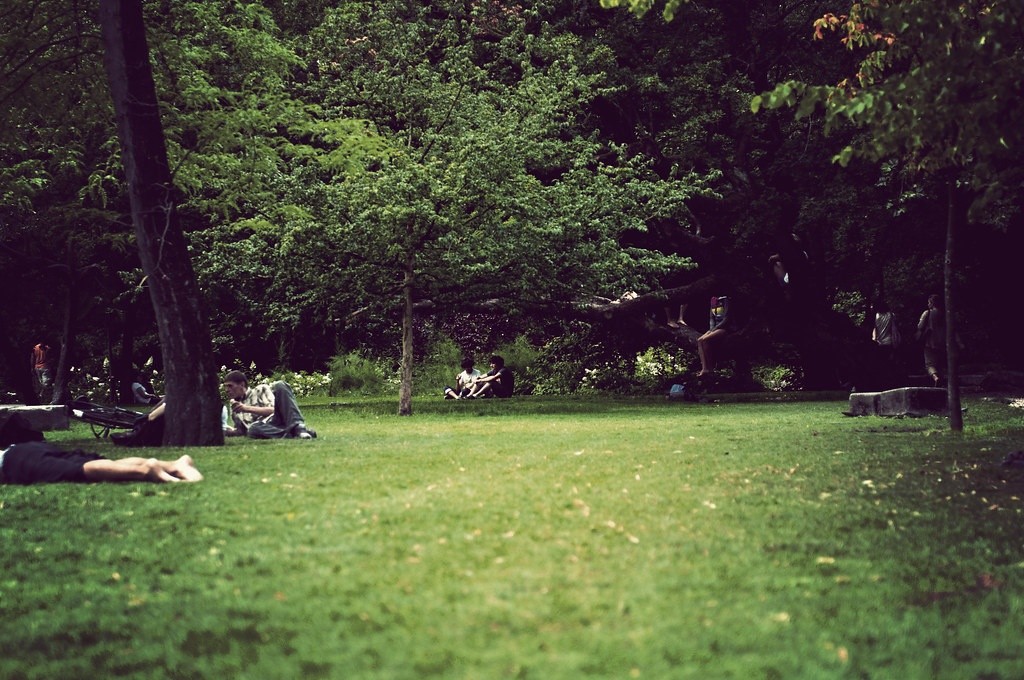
31 337 55 385
110 376 228 444
131 373 162 404
621 286 637 300
872 303 897 359
917 295 964 387
0 442 202 485
697 296 730 377
444 356 514 400
663 278 688 328
768 233 808 283
224 370 316 439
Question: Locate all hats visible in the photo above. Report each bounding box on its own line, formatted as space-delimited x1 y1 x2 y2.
461 356 475 370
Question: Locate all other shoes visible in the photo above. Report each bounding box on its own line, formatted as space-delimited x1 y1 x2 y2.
298 423 318 440
